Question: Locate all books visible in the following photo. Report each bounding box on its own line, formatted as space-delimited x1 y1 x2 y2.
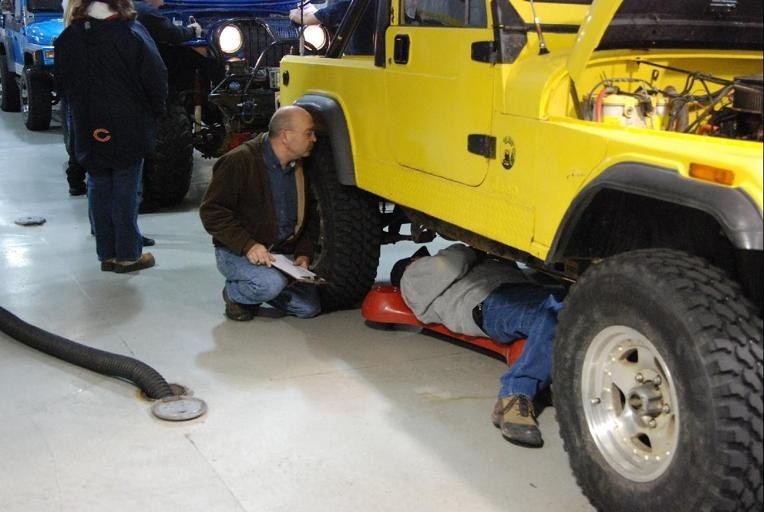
267 253 316 280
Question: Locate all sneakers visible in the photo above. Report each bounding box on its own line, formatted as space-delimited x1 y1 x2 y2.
223 287 254 321
69 182 86 195
141 236 152 247
493 396 544 447
102 252 154 273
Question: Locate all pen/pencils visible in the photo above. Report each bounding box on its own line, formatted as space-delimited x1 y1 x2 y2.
255 244 274 265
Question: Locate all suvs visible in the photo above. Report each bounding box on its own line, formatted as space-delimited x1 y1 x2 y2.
257 1 764 512
0 0 358 215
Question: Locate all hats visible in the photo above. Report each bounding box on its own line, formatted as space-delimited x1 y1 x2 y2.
390 246 430 288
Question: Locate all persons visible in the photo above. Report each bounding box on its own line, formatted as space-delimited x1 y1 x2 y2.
389 243 567 448
198 103 319 321
288 0 377 55
53 0 169 274
60 94 88 196
82 0 203 246
